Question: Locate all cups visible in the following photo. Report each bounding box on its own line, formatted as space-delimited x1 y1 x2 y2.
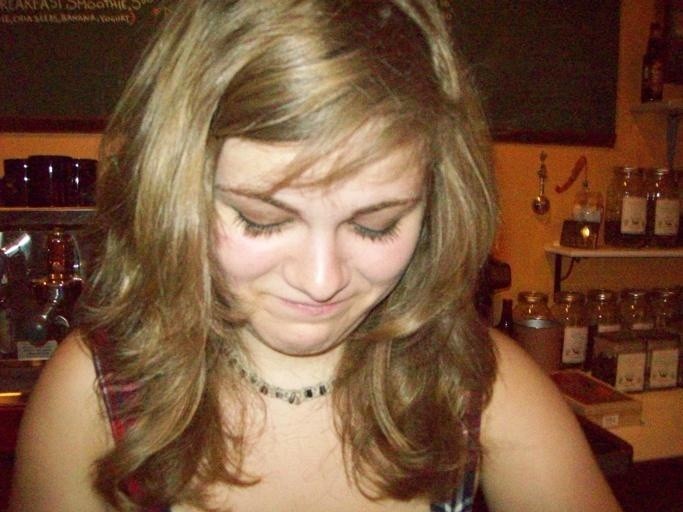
0 153 98 205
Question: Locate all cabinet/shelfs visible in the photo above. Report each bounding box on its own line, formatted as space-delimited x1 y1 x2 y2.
542 97 683 261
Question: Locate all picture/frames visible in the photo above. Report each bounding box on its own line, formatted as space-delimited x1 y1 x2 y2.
435 1 624 151
0 0 183 138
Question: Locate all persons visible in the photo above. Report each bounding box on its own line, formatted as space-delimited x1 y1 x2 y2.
9 0 622 512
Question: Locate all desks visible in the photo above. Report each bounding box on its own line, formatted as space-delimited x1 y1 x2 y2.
547 377 681 511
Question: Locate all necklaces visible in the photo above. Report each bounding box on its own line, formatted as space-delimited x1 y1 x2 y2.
222 346 336 404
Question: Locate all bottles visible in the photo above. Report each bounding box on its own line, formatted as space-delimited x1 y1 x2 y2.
640 21 664 103
605 164 683 247
495 286 679 371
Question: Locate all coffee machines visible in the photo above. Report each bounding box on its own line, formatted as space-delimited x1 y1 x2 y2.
1 209 110 370
